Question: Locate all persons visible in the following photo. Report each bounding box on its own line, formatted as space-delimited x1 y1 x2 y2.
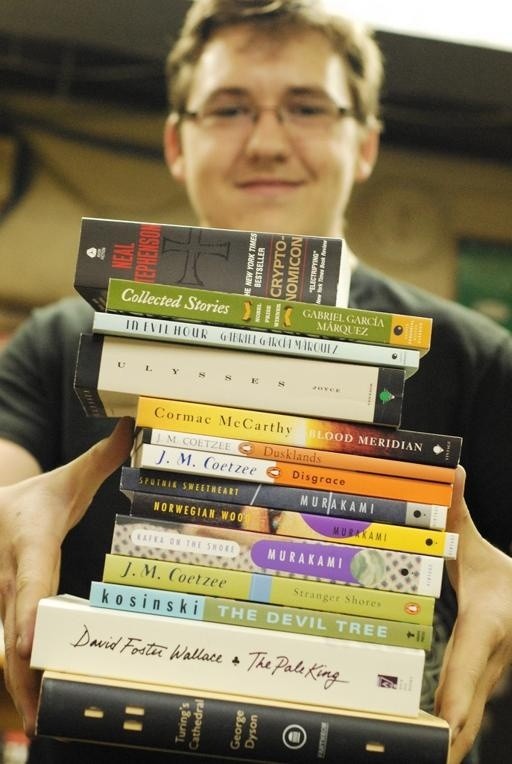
3 0 512 764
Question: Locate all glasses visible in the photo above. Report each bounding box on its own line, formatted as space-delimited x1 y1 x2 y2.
176 103 359 126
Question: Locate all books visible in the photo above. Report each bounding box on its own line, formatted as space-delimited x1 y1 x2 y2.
90 399 464 650
73 217 434 428
30 595 436 718
28 673 450 764
